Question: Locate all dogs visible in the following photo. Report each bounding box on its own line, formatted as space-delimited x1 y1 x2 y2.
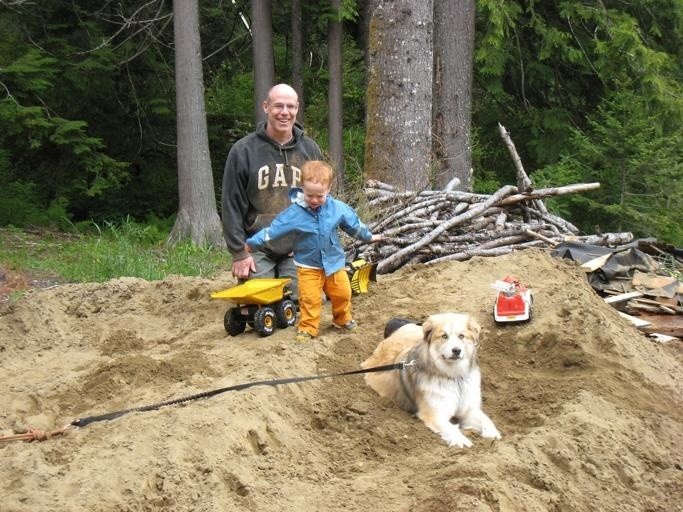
359 313 502 448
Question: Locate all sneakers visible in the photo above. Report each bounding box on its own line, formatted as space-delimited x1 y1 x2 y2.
297 331 311 340
333 319 357 331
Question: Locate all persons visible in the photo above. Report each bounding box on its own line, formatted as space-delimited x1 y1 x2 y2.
221 83 324 312
245 160 382 341
